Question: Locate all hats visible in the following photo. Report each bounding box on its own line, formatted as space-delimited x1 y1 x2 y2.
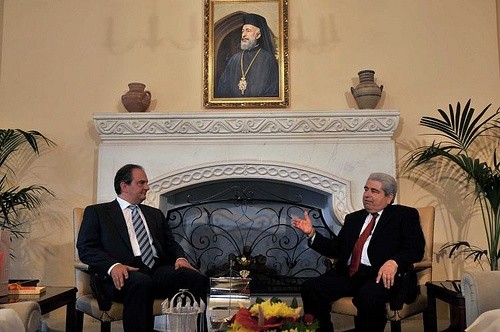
243 14 274 54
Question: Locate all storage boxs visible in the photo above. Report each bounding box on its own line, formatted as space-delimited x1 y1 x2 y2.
8 281 45 294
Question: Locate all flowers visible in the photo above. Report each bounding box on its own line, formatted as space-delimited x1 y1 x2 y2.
227 296 320 332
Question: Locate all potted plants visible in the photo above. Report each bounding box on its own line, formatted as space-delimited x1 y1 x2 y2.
0 129 57 297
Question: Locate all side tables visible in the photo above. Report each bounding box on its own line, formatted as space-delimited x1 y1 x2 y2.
0 286 78 332
426 280 467 332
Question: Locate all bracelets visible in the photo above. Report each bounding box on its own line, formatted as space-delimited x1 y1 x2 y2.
309 230 315 238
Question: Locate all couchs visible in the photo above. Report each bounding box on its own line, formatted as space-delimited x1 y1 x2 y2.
0 301 42 332
461 271 500 332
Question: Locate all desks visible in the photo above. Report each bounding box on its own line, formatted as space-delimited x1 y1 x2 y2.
206 286 251 332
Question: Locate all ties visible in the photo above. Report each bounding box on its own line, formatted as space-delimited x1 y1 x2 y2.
127 205 155 268
349 212 379 277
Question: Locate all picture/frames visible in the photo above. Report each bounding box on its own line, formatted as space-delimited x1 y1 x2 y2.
202 0 290 108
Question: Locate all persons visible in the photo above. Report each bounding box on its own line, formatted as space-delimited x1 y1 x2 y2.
292 172 426 332
77 164 210 332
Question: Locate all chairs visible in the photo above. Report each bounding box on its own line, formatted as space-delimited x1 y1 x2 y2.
73 207 172 332
330 206 436 332
